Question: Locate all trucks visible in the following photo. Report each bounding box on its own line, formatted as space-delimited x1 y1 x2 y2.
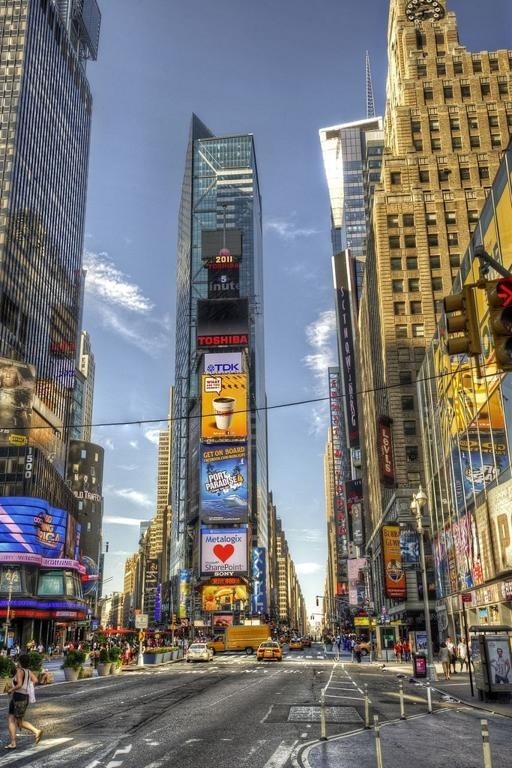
206 625 273 656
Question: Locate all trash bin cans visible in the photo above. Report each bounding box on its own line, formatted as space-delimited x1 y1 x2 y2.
469 625 512 703
414 654 427 677
370 651 376 663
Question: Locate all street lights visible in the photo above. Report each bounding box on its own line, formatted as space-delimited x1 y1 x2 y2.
3 570 20 651
407 482 440 680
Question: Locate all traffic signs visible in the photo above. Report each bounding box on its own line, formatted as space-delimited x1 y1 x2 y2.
461 592 472 602
135 614 149 629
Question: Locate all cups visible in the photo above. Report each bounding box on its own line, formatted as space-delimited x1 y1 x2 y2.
213 396 236 429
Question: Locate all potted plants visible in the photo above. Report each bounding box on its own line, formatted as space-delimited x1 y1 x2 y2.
0 647 123 701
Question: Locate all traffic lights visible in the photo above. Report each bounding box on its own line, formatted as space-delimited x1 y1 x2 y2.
316 598 319 606
313 615 314 620
310 615 312 620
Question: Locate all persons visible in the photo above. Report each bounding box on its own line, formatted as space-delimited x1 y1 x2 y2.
491 647 510 684
4 653 42 750
4 634 475 681
0 365 31 438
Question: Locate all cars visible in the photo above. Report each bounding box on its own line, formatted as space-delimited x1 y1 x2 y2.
256 637 282 662
276 628 316 651
359 639 377 657
106 636 211 650
186 642 214 663
